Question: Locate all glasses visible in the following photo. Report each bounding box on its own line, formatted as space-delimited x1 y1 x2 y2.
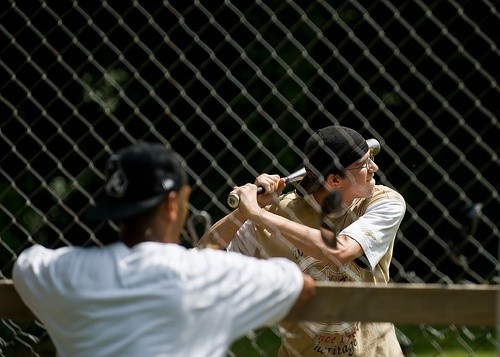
343 153 375 171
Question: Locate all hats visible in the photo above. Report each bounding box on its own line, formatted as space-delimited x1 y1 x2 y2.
88 144 187 219
296 126 369 198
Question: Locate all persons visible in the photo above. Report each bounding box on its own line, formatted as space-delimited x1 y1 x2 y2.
10 142 318 357
195 126 407 357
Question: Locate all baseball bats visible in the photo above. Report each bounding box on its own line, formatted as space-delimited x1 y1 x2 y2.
227 138 381 209
